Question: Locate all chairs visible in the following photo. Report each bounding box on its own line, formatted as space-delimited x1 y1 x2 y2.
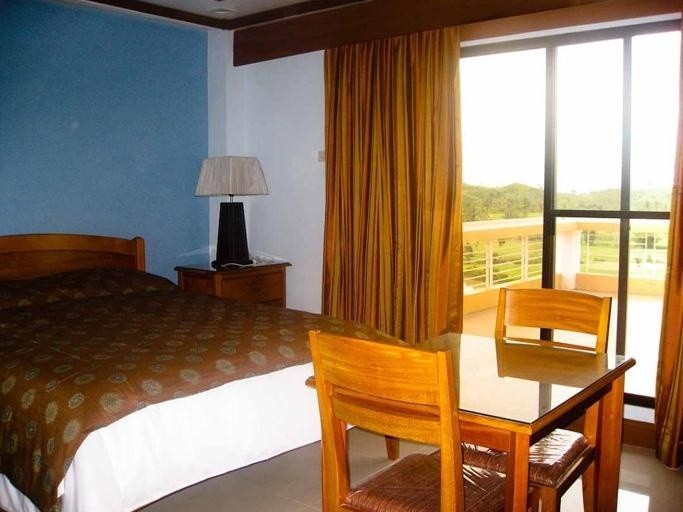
309 330 540 512
429 288 612 512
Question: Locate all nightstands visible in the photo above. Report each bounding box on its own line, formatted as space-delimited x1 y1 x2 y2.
174 263 290 308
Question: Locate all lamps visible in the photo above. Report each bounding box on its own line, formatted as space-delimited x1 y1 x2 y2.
193 156 269 268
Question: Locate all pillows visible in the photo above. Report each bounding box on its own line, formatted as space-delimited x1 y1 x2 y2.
0 267 177 312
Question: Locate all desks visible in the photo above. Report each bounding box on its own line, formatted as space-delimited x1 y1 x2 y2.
306 334 636 512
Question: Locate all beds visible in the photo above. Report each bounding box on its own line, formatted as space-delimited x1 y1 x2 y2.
0 234 410 512
